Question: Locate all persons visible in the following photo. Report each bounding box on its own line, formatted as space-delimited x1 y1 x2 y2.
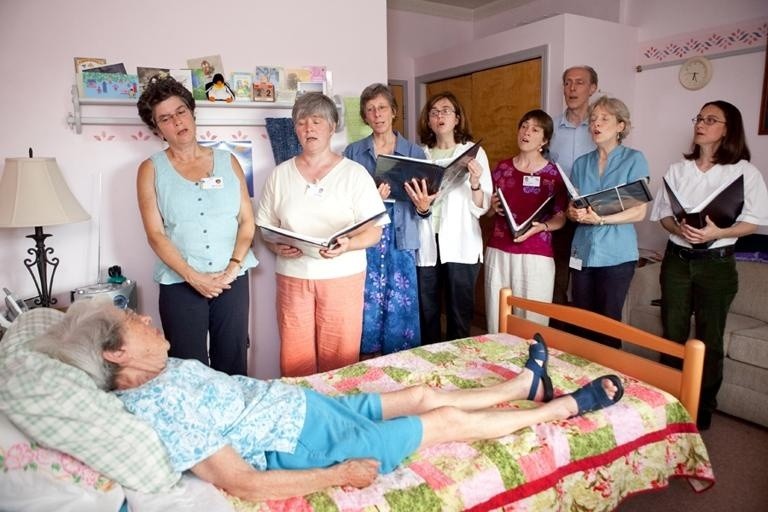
650 101 768 432
484 65 650 349
17 294 623 504
253 92 391 378
341 83 493 363
136 74 259 375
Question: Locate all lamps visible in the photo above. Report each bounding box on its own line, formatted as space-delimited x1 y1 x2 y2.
0 147 91 306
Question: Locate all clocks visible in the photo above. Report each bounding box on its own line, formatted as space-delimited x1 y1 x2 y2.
680 56 711 90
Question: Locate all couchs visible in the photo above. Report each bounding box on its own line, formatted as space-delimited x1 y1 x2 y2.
621 261 768 428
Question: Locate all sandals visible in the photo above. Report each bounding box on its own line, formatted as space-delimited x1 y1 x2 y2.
524 333 554 403
556 375 623 419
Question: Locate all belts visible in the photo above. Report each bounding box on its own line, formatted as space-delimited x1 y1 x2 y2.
668 242 735 258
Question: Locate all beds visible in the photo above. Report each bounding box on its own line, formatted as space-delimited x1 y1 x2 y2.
0 287 705 512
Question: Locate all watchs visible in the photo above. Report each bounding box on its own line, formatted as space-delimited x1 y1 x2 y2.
230 258 244 269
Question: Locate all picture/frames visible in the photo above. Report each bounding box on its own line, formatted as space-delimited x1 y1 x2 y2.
74 57 106 73
297 82 326 99
233 71 253 101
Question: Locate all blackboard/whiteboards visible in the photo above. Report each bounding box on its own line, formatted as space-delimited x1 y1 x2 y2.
634 44 767 235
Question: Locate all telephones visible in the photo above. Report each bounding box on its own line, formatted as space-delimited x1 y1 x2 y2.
0 287 29 334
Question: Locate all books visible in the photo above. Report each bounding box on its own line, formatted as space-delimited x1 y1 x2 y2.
137 54 332 101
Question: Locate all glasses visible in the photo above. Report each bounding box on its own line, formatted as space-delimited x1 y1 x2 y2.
157 105 190 125
692 117 727 125
428 109 456 117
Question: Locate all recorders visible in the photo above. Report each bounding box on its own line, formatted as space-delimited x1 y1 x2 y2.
70 279 137 311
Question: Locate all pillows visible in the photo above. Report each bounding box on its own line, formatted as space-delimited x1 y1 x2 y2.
0 308 182 492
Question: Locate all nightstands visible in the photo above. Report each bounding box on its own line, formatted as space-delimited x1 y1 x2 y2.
0 292 72 340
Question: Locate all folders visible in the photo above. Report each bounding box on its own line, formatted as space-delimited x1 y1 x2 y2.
497 187 557 239
552 158 653 217
375 137 485 206
